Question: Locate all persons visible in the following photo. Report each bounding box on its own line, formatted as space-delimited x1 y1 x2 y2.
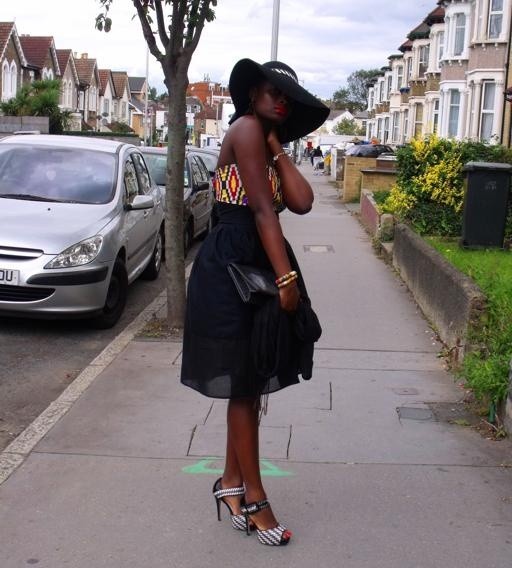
172 57 334 547
302 145 323 165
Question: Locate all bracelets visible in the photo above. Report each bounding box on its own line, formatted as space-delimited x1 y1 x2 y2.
278 274 298 288
271 151 288 161
274 270 297 284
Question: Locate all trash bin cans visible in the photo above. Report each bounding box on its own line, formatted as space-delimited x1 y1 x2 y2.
459 161 512 251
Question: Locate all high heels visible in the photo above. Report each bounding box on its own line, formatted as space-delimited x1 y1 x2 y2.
212 476 255 531
239 496 291 545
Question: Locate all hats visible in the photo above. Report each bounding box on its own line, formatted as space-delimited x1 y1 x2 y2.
228 58 330 146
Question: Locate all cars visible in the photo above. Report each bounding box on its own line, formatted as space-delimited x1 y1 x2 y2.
282 148 293 161
312 143 396 170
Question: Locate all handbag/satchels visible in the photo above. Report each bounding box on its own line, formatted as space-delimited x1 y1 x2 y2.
227 261 282 305
